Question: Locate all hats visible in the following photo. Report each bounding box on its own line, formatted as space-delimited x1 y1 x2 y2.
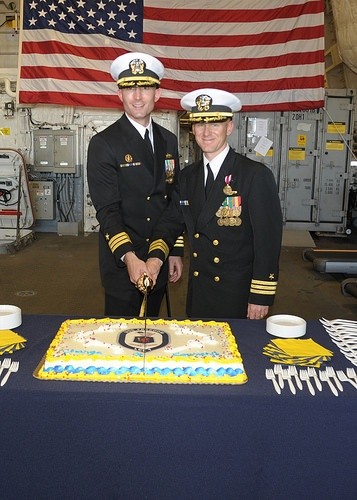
110 52 165 89
180 87 242 123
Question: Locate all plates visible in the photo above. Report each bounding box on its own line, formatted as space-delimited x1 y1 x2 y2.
266 315 306 338
0 305 22 330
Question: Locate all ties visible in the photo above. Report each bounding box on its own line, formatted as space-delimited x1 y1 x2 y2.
205 163 214 200
143 129 154 155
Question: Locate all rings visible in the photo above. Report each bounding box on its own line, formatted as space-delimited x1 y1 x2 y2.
261 315 266 317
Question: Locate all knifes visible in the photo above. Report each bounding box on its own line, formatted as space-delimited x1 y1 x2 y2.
143 276 151 362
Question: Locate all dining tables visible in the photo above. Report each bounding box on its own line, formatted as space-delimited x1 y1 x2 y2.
0 316 357 500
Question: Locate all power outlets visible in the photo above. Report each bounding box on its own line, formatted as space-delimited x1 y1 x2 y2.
6 12 17 31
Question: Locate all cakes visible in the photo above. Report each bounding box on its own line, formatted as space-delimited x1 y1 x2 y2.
38 318 247 384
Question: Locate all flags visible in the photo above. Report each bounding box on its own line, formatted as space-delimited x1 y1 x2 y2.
16 0 325 111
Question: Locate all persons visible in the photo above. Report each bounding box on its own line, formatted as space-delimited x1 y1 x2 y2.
146 88 282 319
87 52 184 317
